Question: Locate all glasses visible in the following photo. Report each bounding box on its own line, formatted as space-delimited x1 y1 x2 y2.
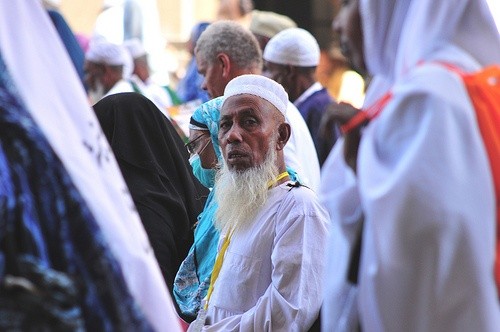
184 133 209 154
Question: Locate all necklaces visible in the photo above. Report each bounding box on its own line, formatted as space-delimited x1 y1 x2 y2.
204 172 291 315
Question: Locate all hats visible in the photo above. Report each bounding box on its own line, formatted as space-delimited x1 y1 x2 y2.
84 37 125 65
122 48 135 79
192 22 210 46
120 39 147 59
221 74 288 120
189 96 225 169
249 10 296 39
263 28 320 66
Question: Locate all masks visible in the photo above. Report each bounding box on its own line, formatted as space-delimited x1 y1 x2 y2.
189 153 220 187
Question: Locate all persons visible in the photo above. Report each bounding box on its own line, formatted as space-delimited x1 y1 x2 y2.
0 1 499 332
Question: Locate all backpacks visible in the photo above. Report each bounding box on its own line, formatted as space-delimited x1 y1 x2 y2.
340 59 500 302
